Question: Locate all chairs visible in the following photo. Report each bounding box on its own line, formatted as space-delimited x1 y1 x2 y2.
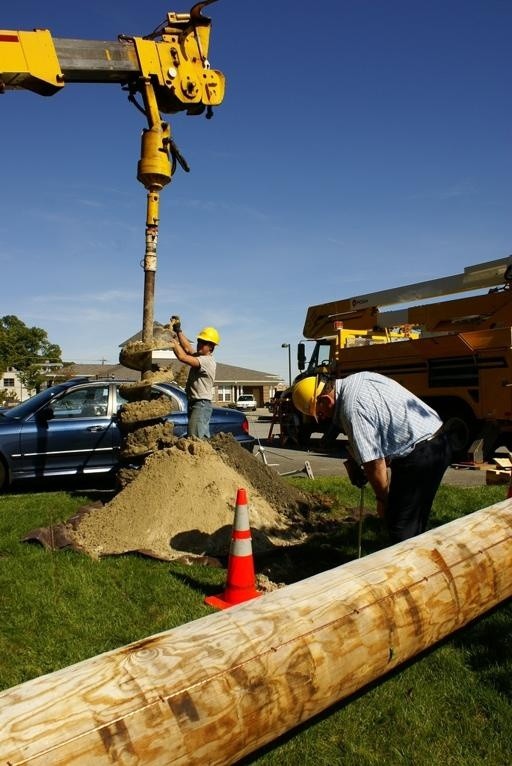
82 399 105 415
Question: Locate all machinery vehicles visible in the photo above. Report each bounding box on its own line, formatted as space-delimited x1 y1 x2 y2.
280 254 512 464
0 0 226 489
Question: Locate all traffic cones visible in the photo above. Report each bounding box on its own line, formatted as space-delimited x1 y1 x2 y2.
203 488 263 611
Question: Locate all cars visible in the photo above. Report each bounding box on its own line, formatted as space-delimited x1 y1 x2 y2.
236 394 257 411
0 377 255 495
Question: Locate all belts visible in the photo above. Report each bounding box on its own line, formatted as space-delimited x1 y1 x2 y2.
416 422 448 448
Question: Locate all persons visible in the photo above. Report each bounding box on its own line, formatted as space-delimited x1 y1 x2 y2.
290 370 452 543
169 315 221 439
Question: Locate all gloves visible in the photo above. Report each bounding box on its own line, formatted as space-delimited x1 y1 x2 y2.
164 315 182 337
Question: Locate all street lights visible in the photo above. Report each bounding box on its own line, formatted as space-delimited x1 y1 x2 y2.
281 343 292 387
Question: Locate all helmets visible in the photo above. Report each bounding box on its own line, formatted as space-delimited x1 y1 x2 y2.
292 373 326 423
197 327 220 345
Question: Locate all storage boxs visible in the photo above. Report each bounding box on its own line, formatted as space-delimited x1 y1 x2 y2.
482 457 511 485
